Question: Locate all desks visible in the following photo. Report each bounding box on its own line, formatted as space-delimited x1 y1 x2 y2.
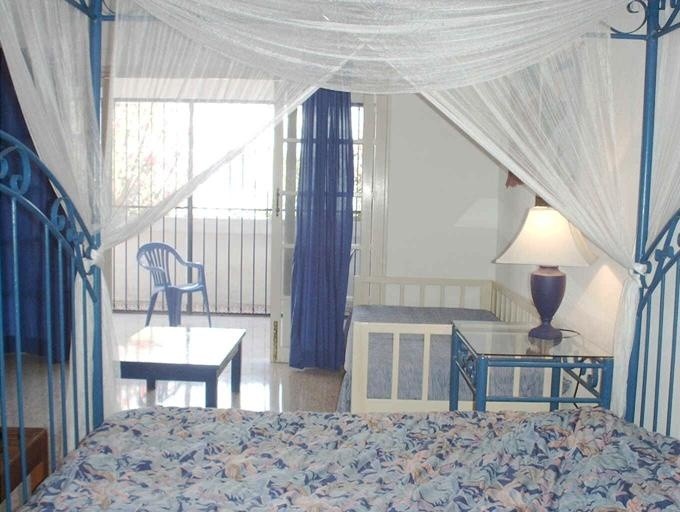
119 327 247 407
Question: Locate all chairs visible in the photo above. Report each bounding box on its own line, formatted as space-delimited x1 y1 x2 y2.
138 241 217 327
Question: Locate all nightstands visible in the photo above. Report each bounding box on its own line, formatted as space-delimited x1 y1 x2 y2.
448 321 614 409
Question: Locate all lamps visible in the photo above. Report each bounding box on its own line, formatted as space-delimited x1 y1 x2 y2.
493 206 601 341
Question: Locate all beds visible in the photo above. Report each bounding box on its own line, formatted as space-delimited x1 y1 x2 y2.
336 276 563 412
1 127 679 507
